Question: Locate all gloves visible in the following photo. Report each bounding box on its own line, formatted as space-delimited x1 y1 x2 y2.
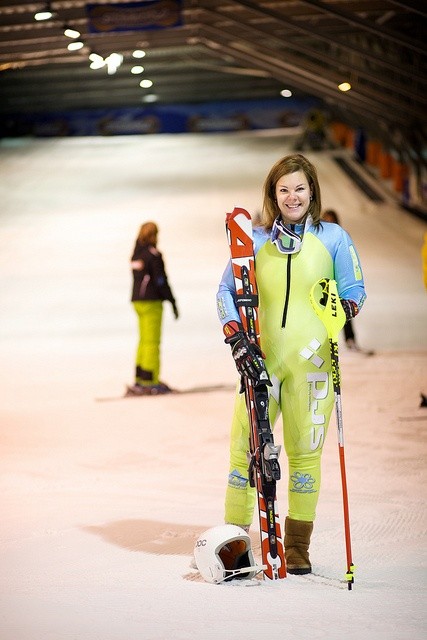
223 332 266 380
340 298 359 320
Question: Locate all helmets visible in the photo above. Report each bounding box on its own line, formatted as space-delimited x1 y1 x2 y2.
194 524 268 584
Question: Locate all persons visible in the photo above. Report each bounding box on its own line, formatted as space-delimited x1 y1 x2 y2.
130 222 179 393
214 154 367 576
321 208 359 352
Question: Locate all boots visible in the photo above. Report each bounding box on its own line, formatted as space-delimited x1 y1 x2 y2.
283 515 313 575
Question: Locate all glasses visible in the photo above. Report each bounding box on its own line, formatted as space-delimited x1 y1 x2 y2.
270 213 303 255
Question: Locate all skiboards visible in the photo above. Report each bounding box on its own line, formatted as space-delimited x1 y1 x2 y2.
347 342 377 358
95 377 227 403
225 208 290 582
